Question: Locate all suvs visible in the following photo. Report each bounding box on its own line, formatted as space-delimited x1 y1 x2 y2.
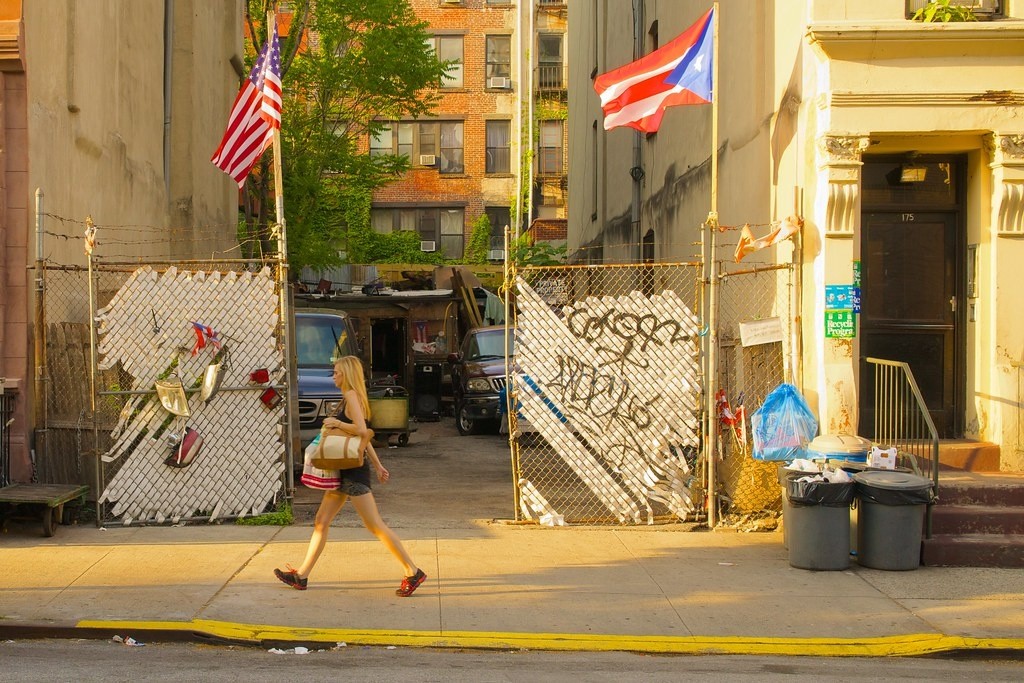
292 306 362 470
449 323 516 433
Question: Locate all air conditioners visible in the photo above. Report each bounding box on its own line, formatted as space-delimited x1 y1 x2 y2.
491 250 503 259
421 241 436 251
491 77 505 87
419 155 435 165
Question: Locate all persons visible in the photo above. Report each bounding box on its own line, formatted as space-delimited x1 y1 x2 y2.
274 355 427 596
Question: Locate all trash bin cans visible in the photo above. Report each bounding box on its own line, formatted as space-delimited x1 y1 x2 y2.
412 361 442 422
808 433 873 466
841 462 936 571
778 464 854 570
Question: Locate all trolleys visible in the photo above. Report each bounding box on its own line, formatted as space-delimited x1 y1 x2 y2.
374 428 419 446
0 482 90 537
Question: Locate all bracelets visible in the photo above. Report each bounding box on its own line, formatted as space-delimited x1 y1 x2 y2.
338 421 342 428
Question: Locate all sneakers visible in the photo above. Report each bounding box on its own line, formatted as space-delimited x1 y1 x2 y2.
274 564 308 590
396 568 428 597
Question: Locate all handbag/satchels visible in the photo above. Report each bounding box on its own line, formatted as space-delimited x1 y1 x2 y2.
300 432 341 491
311 397 374 470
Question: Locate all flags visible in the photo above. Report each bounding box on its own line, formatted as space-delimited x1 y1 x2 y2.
412 321 429 344
592 3 711 135
209 15 285 191
